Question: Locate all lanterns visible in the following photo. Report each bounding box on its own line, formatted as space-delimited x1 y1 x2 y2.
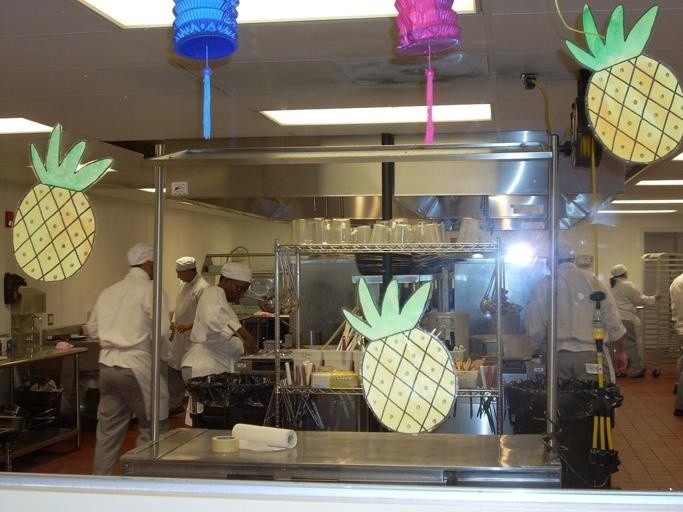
394 0 462 145
171 0 240 141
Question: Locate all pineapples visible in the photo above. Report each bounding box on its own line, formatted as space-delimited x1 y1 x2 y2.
565 4 683 166
12 124 116 282
341 277 460 433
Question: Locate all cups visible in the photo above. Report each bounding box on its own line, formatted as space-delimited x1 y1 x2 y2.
291 217 493 243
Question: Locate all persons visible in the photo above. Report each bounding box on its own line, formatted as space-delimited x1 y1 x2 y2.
610 264 662 378
86 241 171 476
181 261 258 426
523 242 629 383
167 256 210 414
669 274 683 415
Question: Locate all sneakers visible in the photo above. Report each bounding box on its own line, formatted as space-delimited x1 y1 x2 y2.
170 407 185 418
617 369 645 378
674 409 683 416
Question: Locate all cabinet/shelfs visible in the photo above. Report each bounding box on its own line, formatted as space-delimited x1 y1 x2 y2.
275 238 502 435
0 346 89 464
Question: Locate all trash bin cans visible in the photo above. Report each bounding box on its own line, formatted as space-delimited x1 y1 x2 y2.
183 372 285 431
504 376 623 489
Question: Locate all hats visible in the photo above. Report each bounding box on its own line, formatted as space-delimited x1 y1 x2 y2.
221 263 251 284
577 254 593 266
175 257 195 272
556 242 574 260
128 243 154 266
611 264 626 276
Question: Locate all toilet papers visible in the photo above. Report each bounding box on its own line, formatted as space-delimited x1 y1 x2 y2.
231 423 298 449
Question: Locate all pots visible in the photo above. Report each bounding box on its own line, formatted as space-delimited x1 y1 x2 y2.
424 311 470 362
471 335 534 362
0 384 64 438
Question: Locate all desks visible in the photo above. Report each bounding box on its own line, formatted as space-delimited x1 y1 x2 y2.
122 427 568 486
